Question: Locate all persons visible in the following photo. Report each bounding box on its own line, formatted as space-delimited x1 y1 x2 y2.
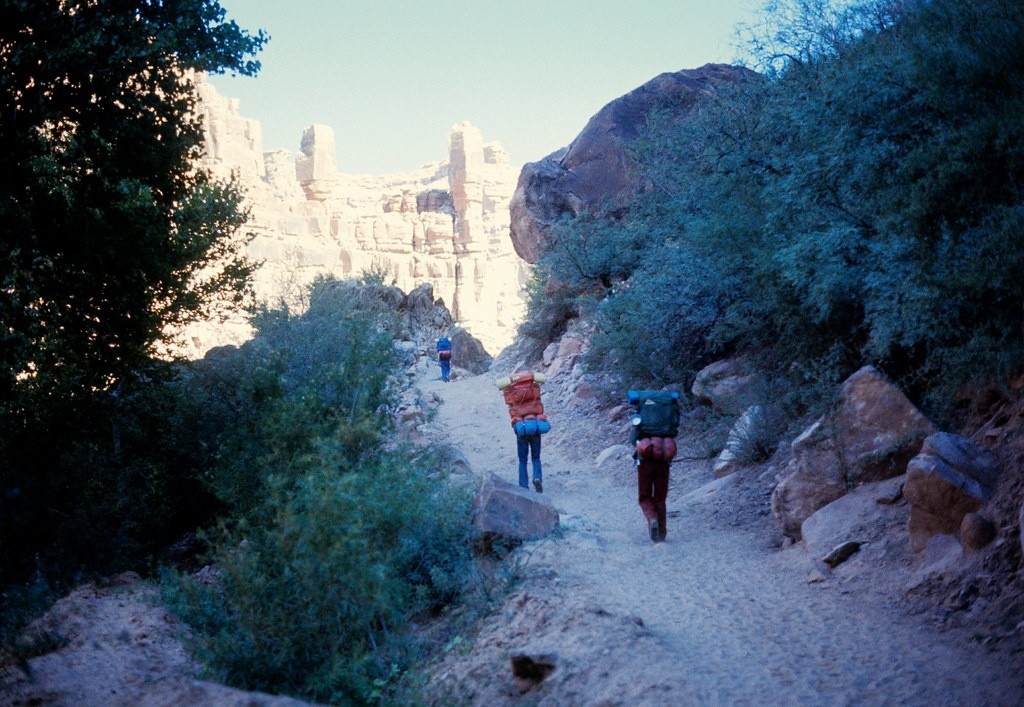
437 343 450 383
517 383 543 492
629 405 673 542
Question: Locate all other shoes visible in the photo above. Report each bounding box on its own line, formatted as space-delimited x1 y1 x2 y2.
532 477 543 494
443 378 450 383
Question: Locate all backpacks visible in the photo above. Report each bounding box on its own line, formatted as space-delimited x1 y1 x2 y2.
496 371 551 436
627 388 681 469
435 337 453 363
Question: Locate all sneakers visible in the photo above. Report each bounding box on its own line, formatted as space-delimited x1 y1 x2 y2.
656 517 666 541
649 519 660 543
